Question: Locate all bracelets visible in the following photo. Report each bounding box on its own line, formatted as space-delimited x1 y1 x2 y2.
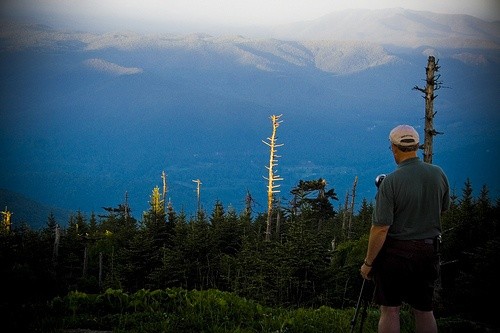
363 259 371 267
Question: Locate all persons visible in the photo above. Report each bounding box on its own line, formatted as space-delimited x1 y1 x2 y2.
360 125 450 333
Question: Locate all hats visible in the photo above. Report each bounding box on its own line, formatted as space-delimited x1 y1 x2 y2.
388 125 420 146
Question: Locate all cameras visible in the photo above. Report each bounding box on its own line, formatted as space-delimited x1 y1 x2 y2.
375 174 387 188
